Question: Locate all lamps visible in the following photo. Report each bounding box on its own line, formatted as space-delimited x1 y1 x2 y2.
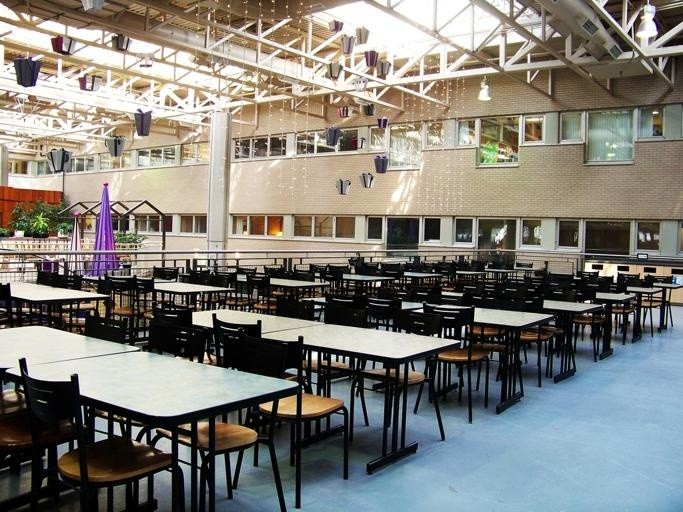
477 64 492 104
634 0 658 39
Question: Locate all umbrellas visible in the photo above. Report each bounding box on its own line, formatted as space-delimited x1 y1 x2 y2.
68 212 84 278
89 183 119 276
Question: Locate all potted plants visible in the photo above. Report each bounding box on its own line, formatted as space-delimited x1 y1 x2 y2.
0 198 75 238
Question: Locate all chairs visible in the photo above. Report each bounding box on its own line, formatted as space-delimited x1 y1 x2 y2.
0 252 460 511
175 252 682 425
